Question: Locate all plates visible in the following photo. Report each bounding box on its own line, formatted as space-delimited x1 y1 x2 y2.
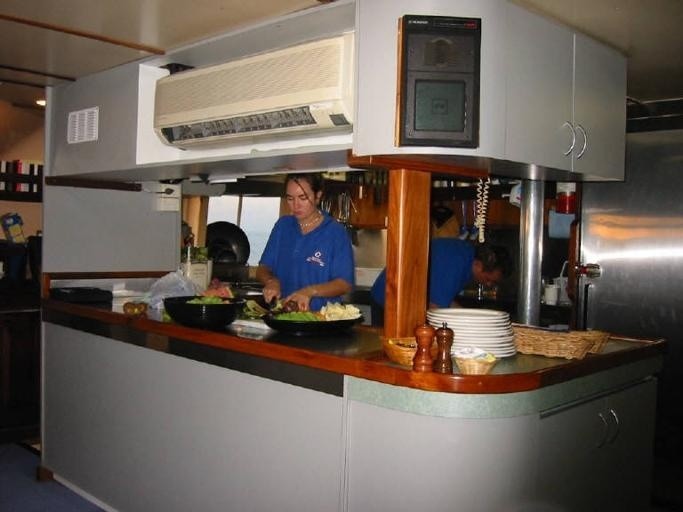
425 309 516 361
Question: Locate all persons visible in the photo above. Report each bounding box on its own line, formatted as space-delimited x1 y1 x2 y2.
371 237 514 329
256 173 355 312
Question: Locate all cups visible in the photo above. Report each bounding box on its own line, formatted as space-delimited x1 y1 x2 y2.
544 277 561 306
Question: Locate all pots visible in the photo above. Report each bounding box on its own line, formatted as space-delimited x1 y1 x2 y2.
205 221 250 266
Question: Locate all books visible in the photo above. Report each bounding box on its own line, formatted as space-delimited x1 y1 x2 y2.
0 158 42 194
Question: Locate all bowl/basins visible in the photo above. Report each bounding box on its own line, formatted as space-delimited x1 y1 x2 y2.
260 310 365 333
162 296 246 328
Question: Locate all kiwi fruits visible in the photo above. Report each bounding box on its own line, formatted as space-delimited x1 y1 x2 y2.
123 302 147 314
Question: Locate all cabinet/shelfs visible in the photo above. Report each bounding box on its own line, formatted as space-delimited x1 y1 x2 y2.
539 375 658 510
504 0 629 183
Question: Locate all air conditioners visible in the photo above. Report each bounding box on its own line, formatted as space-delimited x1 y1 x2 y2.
152 31 352 148
